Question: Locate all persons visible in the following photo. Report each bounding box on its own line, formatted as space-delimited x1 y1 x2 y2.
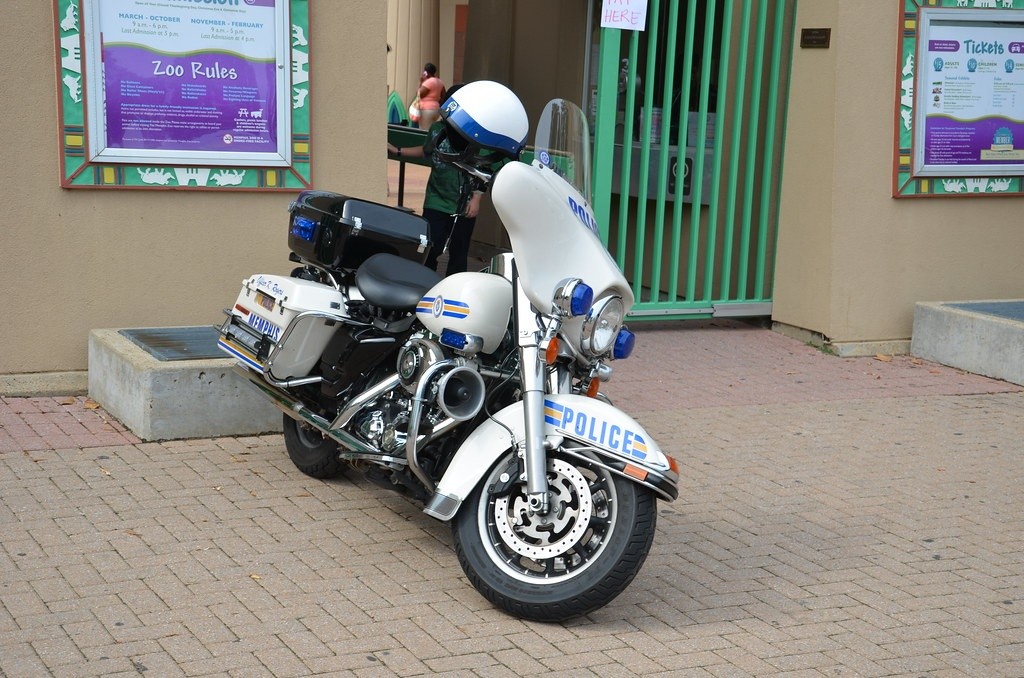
388 83 494 277
410 62 448 133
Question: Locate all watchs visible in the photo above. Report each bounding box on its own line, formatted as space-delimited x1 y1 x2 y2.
398 147 401 157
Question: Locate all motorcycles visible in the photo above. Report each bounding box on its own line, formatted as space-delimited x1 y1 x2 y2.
212 79 679 622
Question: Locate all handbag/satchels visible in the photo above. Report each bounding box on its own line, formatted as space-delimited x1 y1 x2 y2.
408 90 421 122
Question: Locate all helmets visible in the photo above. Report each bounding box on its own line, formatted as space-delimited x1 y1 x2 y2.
438 79 529 160
